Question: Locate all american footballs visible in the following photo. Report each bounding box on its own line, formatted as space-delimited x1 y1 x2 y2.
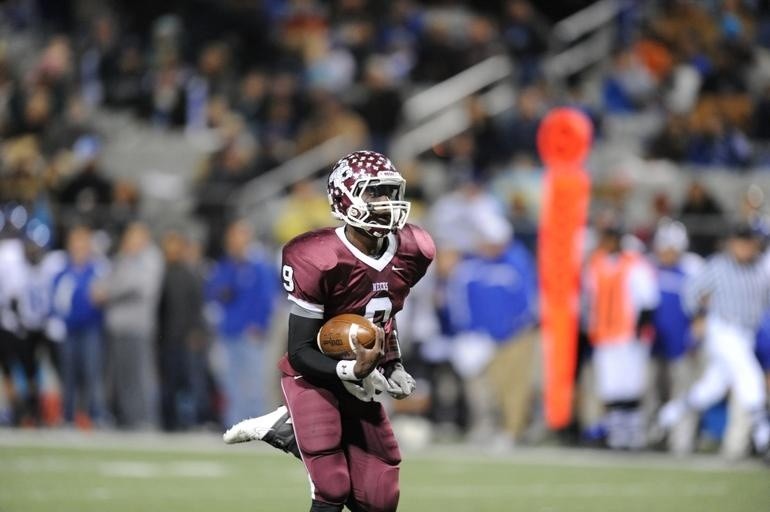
316 314 374 359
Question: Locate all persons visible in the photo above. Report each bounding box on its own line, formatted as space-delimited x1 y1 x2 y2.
0 1 769 462
223 153 435 511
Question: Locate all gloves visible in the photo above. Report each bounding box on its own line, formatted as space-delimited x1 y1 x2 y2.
384 361 417 400
341 367 390 403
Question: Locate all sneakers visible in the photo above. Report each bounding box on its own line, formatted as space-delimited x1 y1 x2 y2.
221 405 290 445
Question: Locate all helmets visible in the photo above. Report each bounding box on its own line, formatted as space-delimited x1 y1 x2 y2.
326 149 408 227
